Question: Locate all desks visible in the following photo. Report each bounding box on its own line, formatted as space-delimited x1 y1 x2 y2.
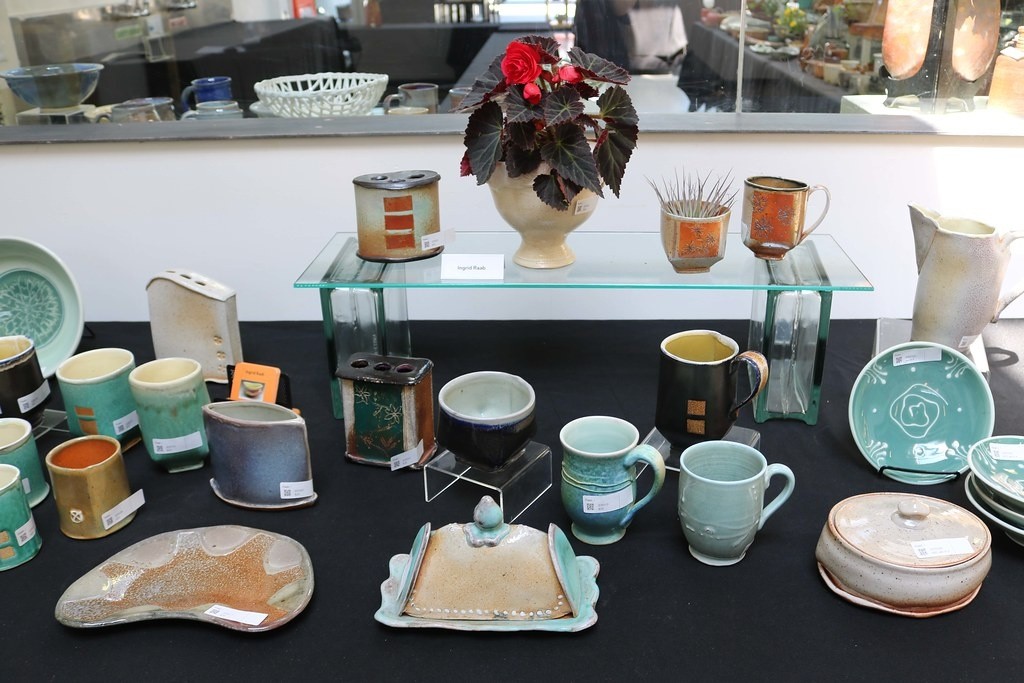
81 16 346 119
675 21 885 113
0 320 1024 683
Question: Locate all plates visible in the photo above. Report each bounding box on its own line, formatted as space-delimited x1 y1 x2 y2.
971 473 1024 525
1 236 85 379
964 471 1024 546
969 435 1024 509
847 341 995 485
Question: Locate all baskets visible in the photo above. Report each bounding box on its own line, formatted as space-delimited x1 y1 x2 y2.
253 73 388 119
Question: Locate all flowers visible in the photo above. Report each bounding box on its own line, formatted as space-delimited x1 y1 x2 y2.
448 34 639 212
775 7 808 43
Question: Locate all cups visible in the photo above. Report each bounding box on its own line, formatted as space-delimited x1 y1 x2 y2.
0 460 42 571
655 329 768 448
0 417 50 508
45 434 136 540
56 348 135 440
741 176 830 261
677 440 795 566
559 415 666 545
383 83 473 115
95 76 244 124
0 335 50 424
438 371 537 471
808 53 884 95
129 357 212 467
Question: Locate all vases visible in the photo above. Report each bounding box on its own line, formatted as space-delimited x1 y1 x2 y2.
487 161 602 269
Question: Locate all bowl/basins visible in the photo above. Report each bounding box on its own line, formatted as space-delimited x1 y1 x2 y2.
840 60 860 69
0 63 104 108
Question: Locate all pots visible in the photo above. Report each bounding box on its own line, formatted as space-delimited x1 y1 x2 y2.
815 492 993 618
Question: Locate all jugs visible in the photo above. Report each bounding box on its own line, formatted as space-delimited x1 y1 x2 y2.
907 200 1024 352
